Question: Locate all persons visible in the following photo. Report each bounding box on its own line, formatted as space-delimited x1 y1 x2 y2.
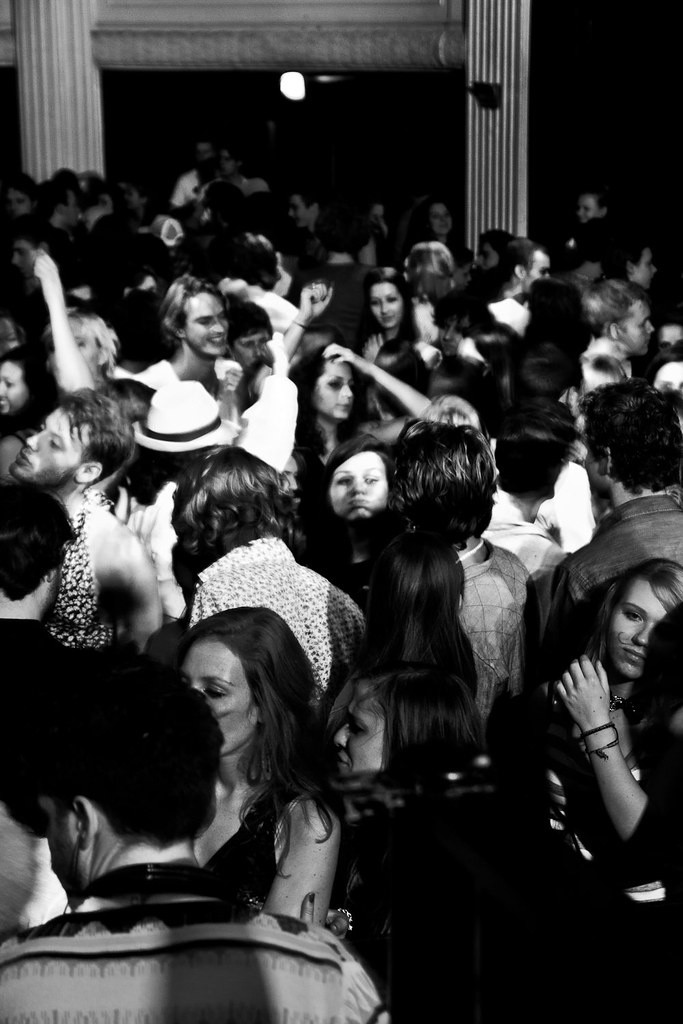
179 607 353 926
0 653 390 1024
0 141 683 1024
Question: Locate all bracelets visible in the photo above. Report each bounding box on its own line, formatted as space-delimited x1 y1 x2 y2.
581 721 615 739
585 727 619 760
337 907 353 932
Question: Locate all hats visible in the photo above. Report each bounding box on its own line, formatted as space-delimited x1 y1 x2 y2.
131 380 228 451
